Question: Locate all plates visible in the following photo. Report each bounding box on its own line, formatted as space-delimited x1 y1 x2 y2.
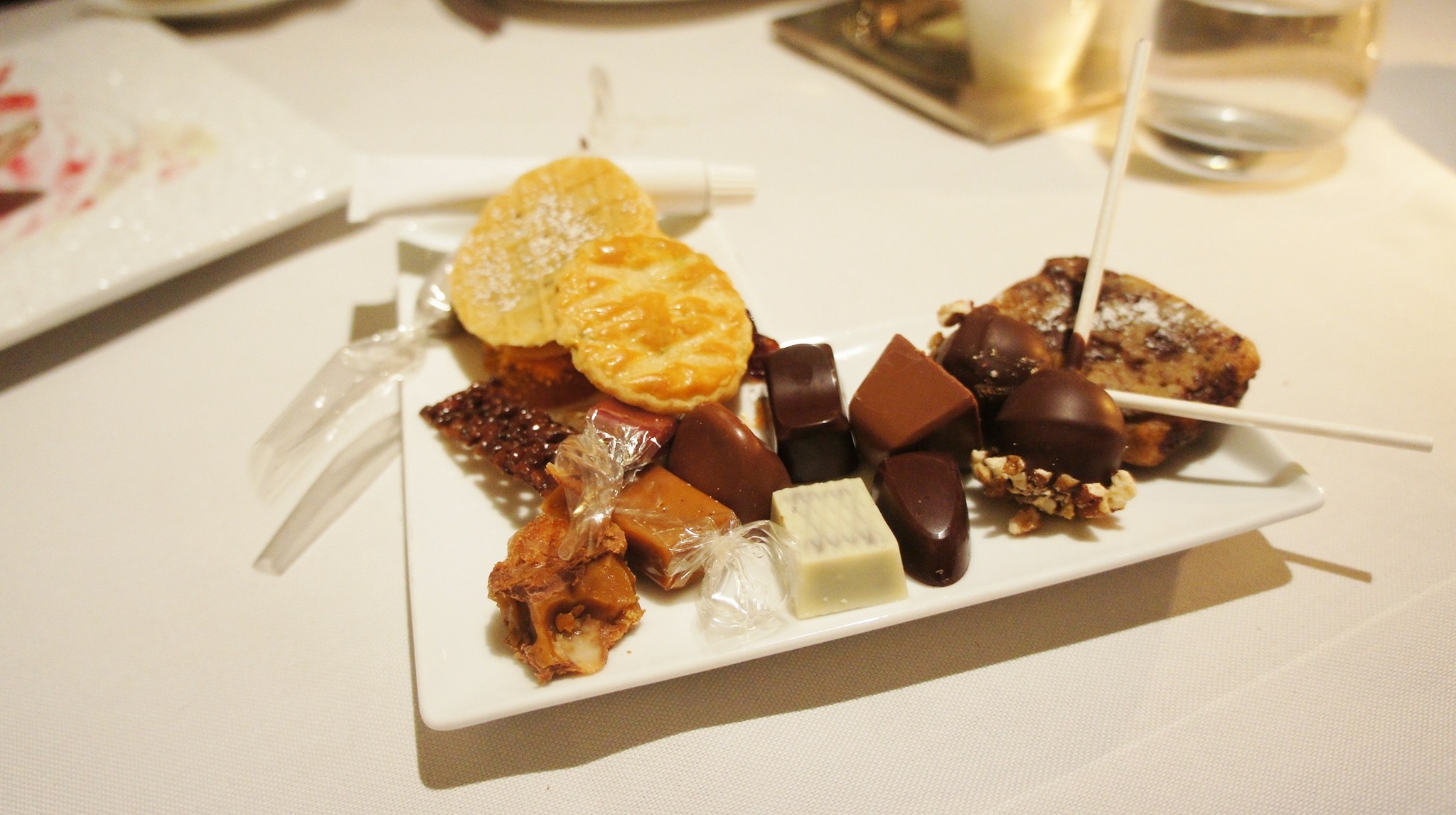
0 0 354 351
772 3 1126 145
400 336 1324 733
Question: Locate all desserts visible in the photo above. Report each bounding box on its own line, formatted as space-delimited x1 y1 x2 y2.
420 157 1260 688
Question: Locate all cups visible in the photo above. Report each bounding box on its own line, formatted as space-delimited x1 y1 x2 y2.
1133 0 1377 185
